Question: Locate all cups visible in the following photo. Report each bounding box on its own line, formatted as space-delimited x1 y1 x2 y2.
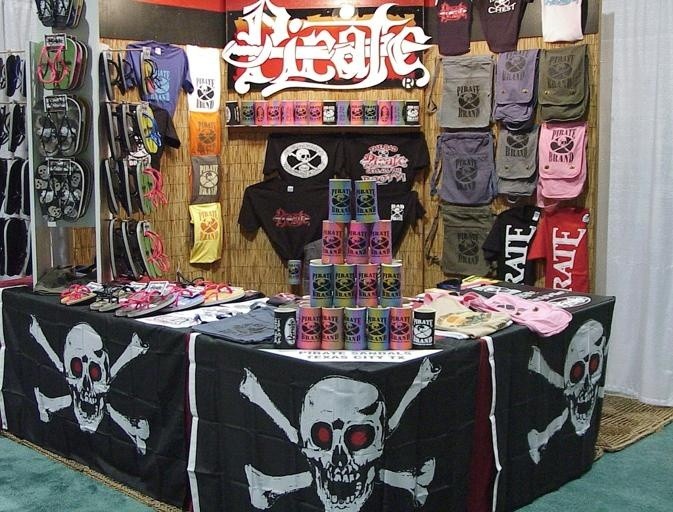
295 100 309 125
309 263 333 307
346 307 367 350
309 100 323 124
336 100 350 125
347 220 369 264
241 100 253 125
329 179 351 223
357 264 378 307
322 308 344 350
355 180 377 223
351 100 364 124
254 100 268 124
363 100 378 125
413 308 436 350
323 100 337 125
392 100 405 124
391 306 413 350
297 305 321 350
322 220 344 264
288 260 302 285
378 100 392 125
226 101 240 124
368 307 391 351
268 101 281 124
333 265 355 307
282 100 294 124
381 263 403 307
371 220 392 264
274 308 296 349
404 100 420 125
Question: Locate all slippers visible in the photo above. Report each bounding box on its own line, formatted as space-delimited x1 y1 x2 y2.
120 104 137 151
31 96 86 158
122 218 146 280
102 158 123 211
8 104 25 152
13 58 26 96
0 105 10 149
118 157 138 216
35 0 84 28
137 161 167 215
4 159 20 215
111 218 129 279
36 38 88 91
35 156 90 223
15 222 32 275
118 52 137 96
137 220 169 280
138 106 163 154
1 54 21 97
61 279 245 317
104 100 121 158
140 51 160 96
16 160 30 215
102 51 119 99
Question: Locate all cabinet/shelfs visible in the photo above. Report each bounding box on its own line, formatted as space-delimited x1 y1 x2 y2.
0 276 617 512
